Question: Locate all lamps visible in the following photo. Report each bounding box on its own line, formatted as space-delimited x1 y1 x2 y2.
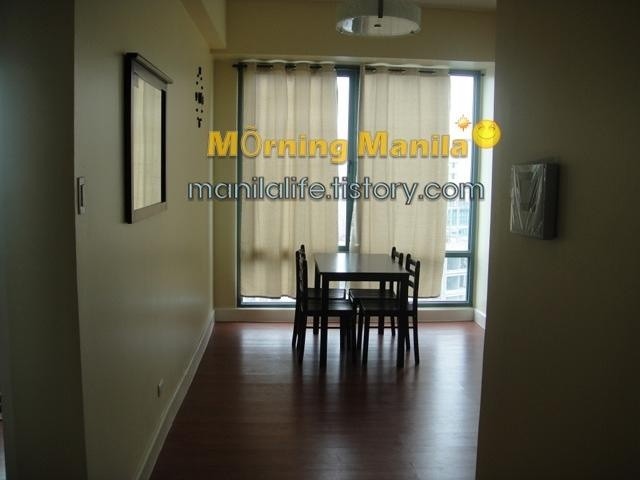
331 0 424 39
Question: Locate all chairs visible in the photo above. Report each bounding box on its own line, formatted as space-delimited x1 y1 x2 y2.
289 243 425 374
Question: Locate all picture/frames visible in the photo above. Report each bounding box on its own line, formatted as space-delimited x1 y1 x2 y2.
123 49 174 224
